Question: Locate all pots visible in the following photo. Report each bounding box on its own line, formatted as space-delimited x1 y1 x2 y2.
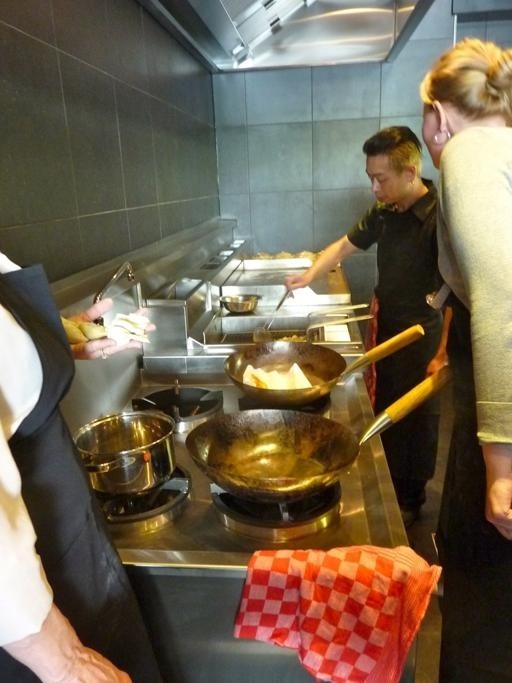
224 322 426 404
185 377 433 505
72 408 176 495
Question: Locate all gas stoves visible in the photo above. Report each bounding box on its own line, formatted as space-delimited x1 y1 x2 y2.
86 368 408 570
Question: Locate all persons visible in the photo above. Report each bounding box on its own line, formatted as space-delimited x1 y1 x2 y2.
281 124 445 529
1 252 167 682
418 33 511 683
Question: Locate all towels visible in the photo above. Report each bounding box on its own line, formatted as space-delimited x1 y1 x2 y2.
231 541 442 683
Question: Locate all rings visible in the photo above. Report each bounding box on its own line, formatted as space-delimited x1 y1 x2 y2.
98 346 111 362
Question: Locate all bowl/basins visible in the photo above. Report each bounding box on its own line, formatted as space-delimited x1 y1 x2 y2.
218 292 262 313
